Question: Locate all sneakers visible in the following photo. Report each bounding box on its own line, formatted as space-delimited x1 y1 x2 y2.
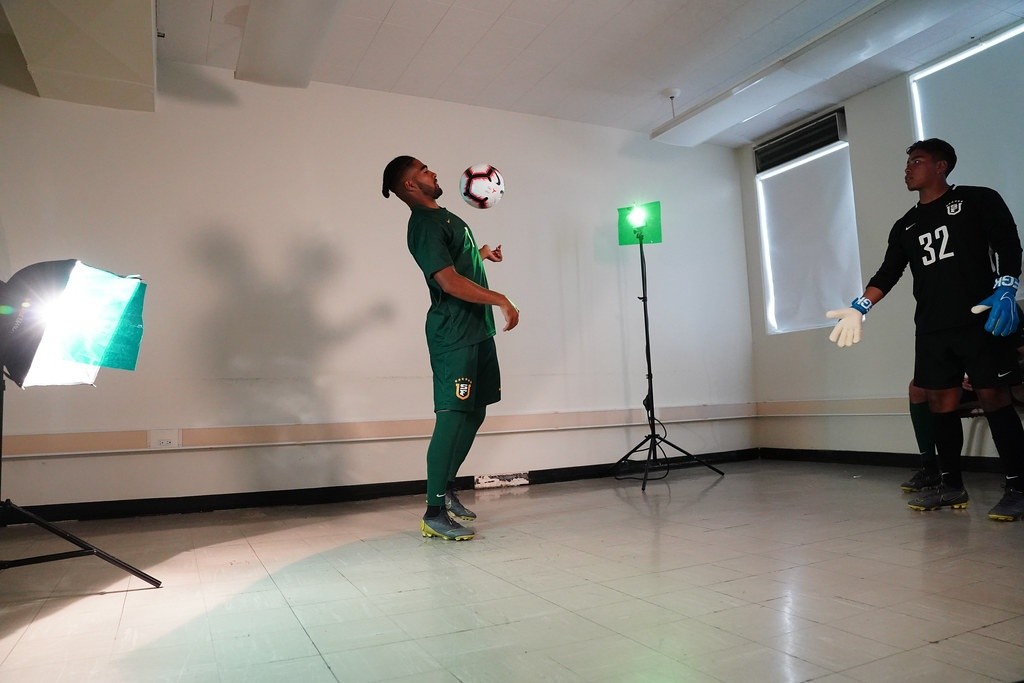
989 488 1024 521
420 489 478 542
901 474 921 493
909 472 969 511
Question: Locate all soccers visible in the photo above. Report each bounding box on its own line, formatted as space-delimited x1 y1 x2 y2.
459 162 506 209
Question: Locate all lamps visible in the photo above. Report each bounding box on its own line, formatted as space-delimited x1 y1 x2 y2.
0 260 162 588
233 1 337 89
649 0 966 147
612 205 726 495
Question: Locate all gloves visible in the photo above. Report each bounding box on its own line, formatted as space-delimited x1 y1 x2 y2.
827 296 873 348
971 276 1020 336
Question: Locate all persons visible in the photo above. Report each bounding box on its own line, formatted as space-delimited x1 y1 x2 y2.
824 138 1024 523
382 156 521 542
900 299 1024 492
